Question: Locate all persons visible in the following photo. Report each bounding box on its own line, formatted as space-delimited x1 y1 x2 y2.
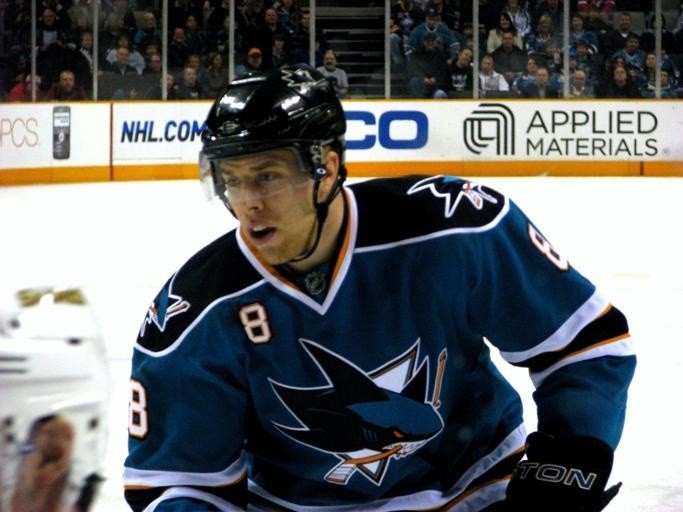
3 0 682 101
122 62 637 511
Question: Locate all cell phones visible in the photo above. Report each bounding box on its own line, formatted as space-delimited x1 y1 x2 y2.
51 106 70 158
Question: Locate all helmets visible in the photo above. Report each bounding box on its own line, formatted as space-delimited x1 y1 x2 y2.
199 67 345 198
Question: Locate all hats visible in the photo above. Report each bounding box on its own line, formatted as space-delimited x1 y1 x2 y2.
428 7 438 17
248 48 262 55
0 288 104 511
